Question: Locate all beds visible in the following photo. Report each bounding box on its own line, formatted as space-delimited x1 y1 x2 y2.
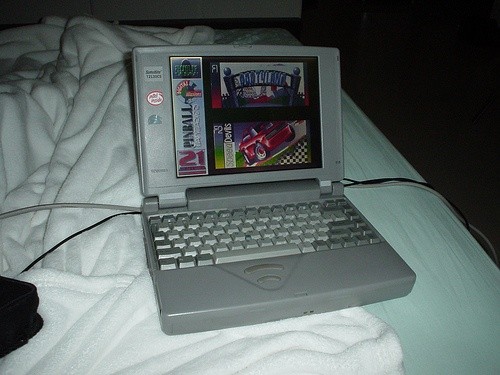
0 0 500 375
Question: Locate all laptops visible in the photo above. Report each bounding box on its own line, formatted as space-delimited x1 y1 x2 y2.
131 46 416 336
